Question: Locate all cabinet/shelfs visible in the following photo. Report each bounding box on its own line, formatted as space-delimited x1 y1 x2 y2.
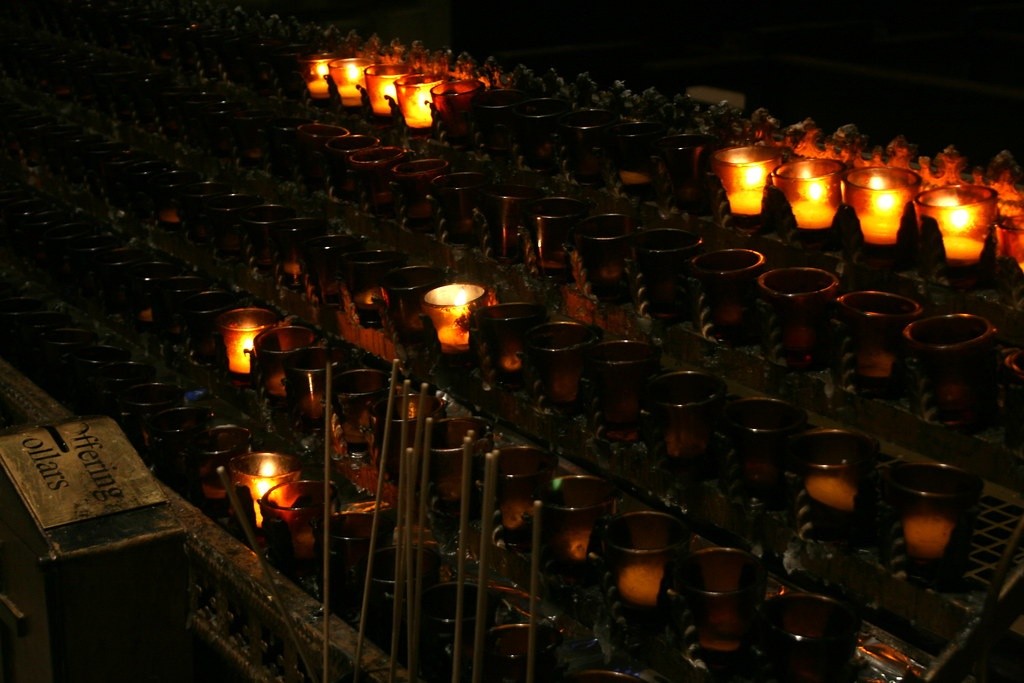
0 1 1023 683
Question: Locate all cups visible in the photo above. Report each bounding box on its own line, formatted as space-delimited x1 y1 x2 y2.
0 0 1024 683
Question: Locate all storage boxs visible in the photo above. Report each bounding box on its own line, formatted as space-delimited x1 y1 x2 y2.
0 418 192 683
